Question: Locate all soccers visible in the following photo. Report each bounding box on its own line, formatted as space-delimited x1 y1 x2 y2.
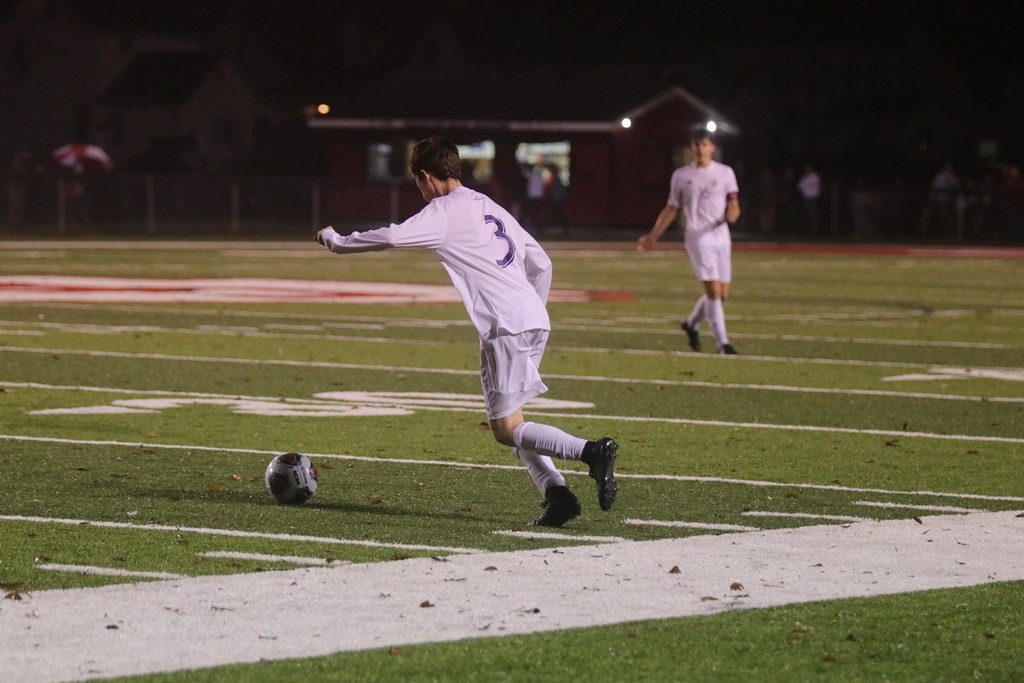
262 452 321 506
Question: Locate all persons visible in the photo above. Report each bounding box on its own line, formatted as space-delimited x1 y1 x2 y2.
0 143 1024 254
638 129 739 355
315 138 619 526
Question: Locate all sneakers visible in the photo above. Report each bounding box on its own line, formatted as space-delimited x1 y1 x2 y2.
718 344 739 354
589 436 620 511
525 491 581 527
680 322 702 350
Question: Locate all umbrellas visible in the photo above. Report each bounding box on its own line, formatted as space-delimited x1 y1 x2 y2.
53 143 114 172
